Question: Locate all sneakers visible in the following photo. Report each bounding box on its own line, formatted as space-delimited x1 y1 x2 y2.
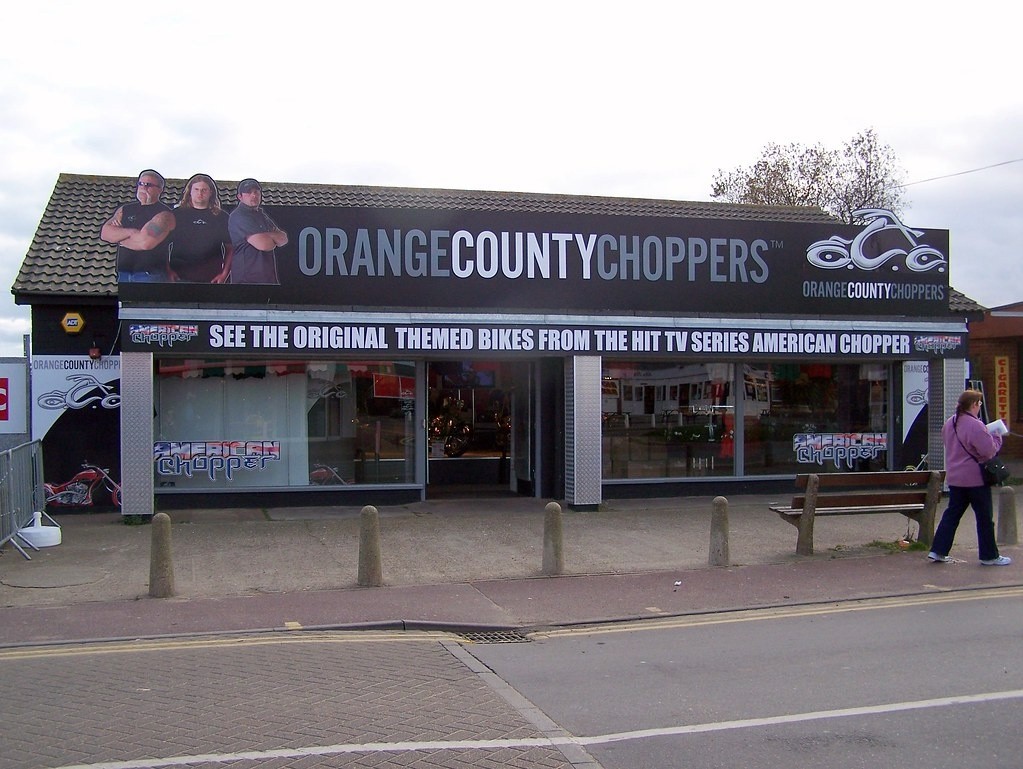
981 555 1012 565
927 552 949 562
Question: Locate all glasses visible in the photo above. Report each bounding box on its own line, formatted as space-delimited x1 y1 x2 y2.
138 180 161 188
977 401 983 405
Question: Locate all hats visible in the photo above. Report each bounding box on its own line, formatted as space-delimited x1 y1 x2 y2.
238 178 261 193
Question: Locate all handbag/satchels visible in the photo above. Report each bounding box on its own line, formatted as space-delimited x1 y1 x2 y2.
980 456 1010 486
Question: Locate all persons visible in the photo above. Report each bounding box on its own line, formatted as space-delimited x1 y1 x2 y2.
100 170 176 284
170 175 232 283
927 390 1012 566
228 179 288 284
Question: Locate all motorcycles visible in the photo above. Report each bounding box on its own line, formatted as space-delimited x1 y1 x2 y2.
37 459 126 513
433 399 513 457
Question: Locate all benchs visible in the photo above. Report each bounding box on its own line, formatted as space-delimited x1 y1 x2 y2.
769 469 946 556
